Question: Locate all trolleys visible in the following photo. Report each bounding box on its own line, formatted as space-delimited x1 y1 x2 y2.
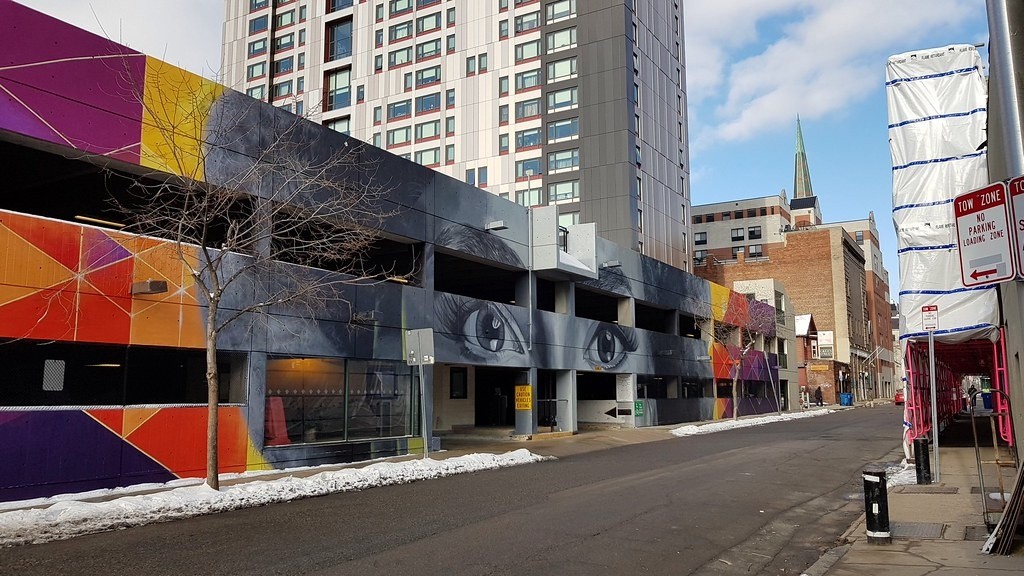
968 387 1022 536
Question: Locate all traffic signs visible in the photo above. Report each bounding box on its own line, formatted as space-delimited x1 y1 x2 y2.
953 181 1018 287
1005 174 1024 280
921 304 938 332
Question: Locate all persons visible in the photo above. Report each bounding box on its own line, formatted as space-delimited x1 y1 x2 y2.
968 385 977 406
814 386 823 407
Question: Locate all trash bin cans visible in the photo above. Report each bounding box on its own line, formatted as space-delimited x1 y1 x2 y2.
839 392 854 406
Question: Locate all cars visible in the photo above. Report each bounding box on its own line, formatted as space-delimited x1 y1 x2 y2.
894 389 904 405
